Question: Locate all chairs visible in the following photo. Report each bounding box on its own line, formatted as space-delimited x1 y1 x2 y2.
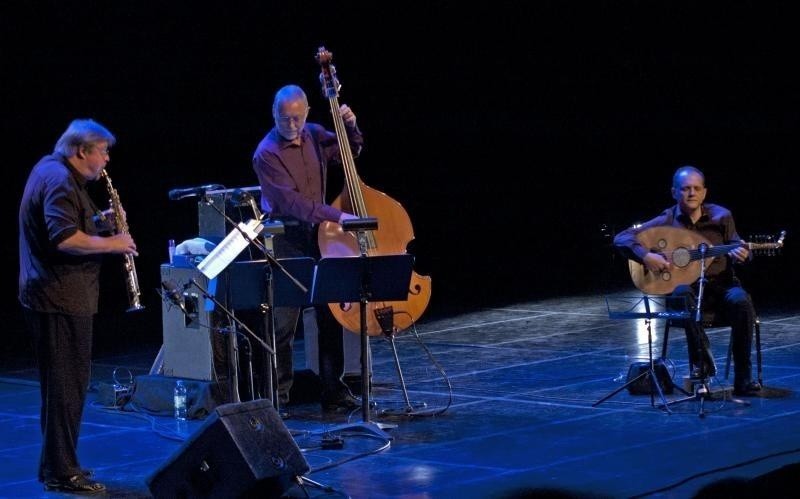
660 285 763 395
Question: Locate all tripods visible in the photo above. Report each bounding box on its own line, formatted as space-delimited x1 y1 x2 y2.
309 302 399 443
590 292 674 417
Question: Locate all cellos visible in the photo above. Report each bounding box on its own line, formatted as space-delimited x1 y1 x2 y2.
314 47 431 337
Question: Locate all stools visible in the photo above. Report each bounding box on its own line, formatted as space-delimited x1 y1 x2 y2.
300 306 374 391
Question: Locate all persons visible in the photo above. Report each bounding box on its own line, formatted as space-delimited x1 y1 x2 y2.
611 165 763 395
17 117 140 496
252 81 377 420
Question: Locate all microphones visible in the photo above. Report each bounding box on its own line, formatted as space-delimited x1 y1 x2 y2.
160 278 189 315
165 181 220 199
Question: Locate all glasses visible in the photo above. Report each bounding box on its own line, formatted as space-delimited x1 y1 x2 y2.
277 114 304 122
95 146 109 156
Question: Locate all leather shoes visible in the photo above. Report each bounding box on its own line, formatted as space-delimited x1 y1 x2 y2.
694 366 716 377
279 409 290 420
38 469 106 494
734 382 761 397
321 388 375 410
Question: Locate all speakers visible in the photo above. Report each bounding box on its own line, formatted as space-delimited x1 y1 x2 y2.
146 398 313 499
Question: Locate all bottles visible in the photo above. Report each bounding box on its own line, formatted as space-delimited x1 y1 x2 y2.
173 380 188 421
168 239 175 263
